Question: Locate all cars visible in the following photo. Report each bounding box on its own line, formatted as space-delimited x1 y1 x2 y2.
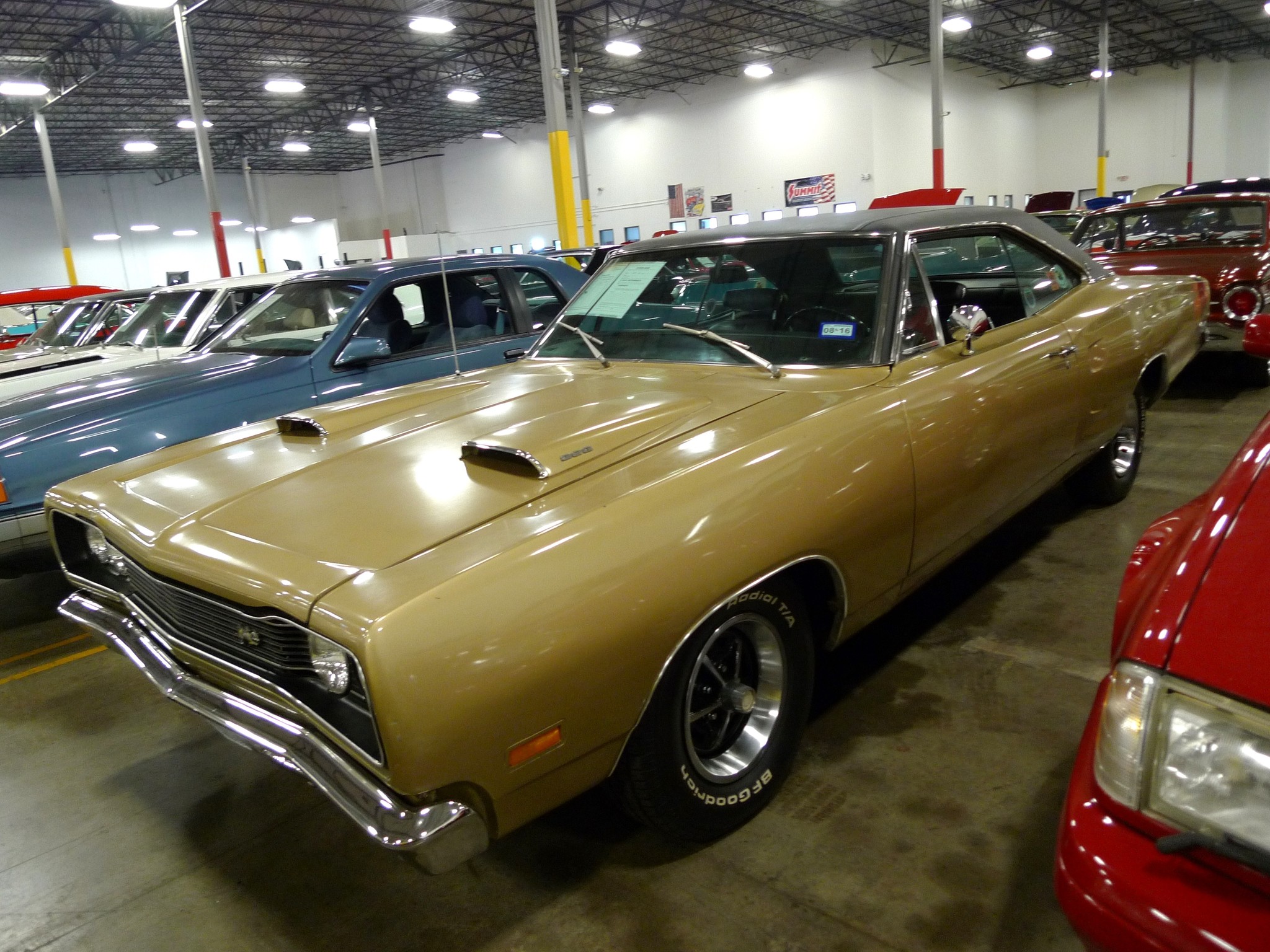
0 175 1270 952
